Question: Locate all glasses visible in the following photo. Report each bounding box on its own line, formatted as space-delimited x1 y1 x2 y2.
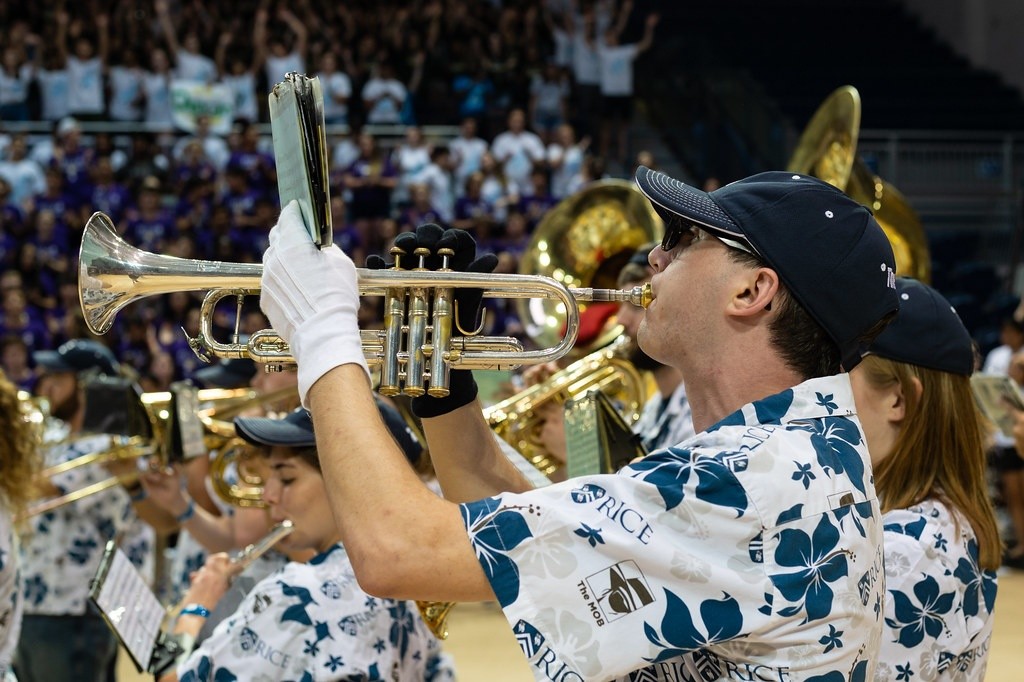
661 214 771 311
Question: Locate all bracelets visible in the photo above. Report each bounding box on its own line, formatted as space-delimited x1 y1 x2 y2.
179 603 211 618
123 480 147 502
175 499 196 523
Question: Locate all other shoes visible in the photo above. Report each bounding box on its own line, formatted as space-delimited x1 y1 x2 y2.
1002 549 1024 569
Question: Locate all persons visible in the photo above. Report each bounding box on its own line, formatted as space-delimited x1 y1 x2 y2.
1 0 1024 682
260 165 897 682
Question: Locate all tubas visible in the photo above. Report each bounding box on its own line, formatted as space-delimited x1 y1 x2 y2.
518 177 667 410
784 84 932 290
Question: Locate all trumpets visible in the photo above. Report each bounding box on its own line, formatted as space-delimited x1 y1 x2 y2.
476 336 644 476
78 209 659 396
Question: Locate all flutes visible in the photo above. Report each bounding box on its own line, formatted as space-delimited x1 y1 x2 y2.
161 520 292 630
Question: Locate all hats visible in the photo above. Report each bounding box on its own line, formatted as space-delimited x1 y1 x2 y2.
234 395 423 467
864 276 975 376
632 240 664 269
33 338 114 373
634 164 901 373
141 176 161 191
197 336 258 388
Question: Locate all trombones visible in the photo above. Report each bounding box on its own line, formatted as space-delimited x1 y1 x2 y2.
7 368 378 521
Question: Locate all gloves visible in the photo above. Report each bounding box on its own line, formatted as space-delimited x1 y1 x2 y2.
366 223 499 418
260 199 374 411
